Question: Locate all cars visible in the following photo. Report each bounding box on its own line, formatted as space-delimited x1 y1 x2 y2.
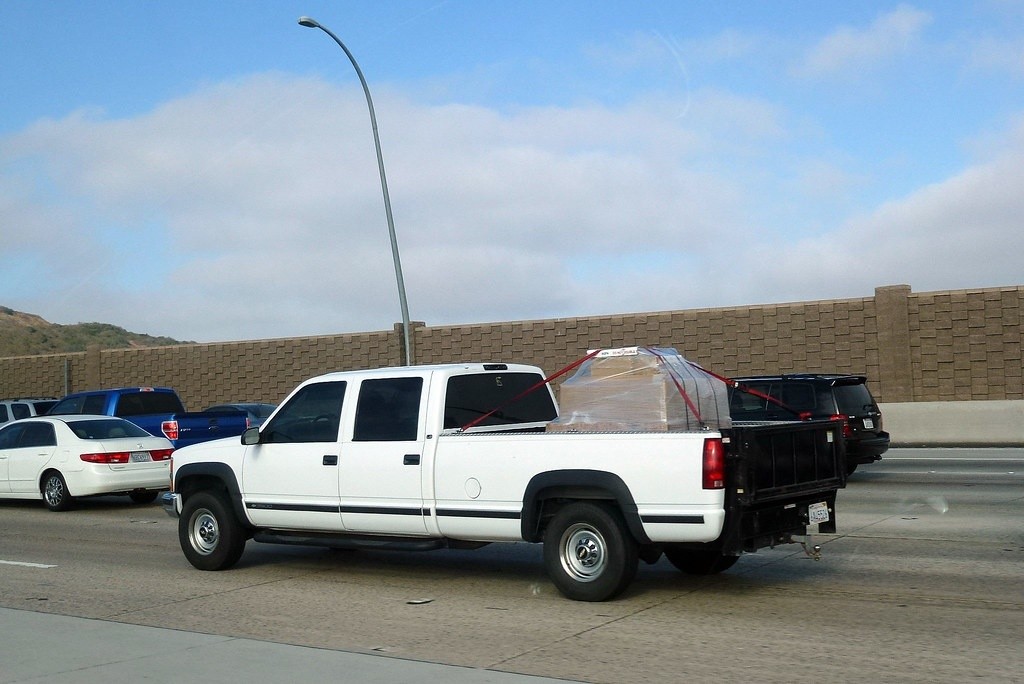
0 414 176 512
202 403 278 428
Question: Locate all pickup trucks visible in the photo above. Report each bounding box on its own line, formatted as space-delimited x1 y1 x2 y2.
161 362 846 602
44 387 250 450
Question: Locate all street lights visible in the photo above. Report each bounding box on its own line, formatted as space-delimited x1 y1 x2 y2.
297 16 410 366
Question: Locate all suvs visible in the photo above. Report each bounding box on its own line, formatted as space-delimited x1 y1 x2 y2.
0 396 61 428
726 372 890 479
